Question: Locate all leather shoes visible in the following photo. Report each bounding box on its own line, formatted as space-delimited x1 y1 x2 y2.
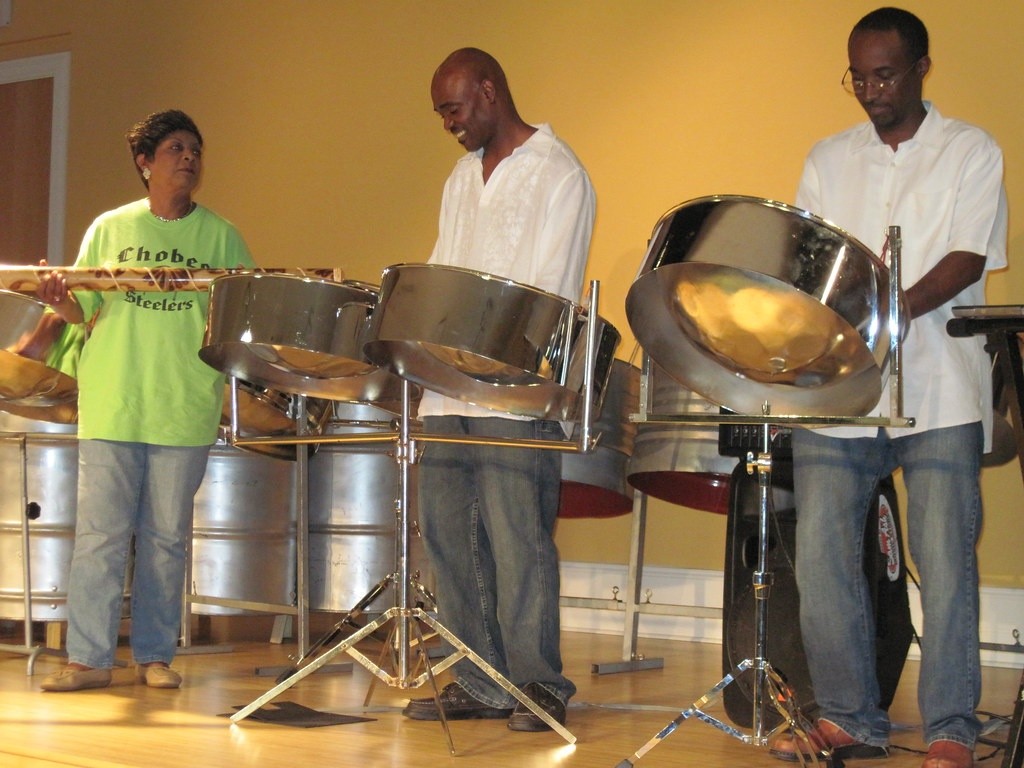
919 739 973 768
508 684 566 732
138 664 182 688
402 682 513 719
769 717 890 761
40 667 112 691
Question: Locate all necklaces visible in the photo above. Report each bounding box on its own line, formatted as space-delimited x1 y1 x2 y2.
145 196 193 222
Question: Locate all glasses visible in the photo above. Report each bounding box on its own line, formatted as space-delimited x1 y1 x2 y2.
842 59 921 99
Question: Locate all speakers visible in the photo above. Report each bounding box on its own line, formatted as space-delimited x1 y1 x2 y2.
722 455 913 733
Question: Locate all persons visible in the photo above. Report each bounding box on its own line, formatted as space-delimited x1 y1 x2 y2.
766 7 1010 768
30 110 257 696
413 46 595 733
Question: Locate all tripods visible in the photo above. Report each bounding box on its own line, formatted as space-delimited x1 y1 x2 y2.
616 402 846 768
233 379 579 749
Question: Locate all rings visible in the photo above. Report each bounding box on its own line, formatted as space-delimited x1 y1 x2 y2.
53 296 60 302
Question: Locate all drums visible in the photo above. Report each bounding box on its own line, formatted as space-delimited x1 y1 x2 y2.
198 272 426 407
623 191 914 429
0 290 87 426
217 372 331 462
363 260 623 425
